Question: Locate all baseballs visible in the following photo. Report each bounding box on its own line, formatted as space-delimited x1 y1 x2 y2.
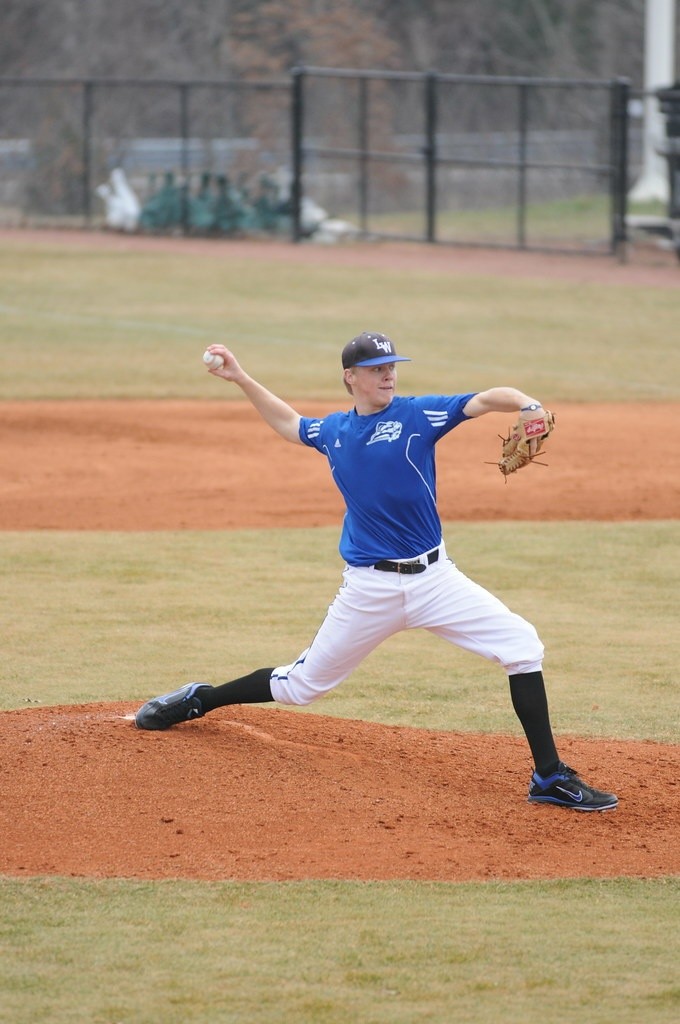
202 349 225 370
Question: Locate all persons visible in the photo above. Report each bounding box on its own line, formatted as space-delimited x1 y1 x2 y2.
134 332 619 810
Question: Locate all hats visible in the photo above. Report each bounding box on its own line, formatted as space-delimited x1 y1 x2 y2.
342 332 412 371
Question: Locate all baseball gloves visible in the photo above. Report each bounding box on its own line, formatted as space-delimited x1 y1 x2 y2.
498 410 555 474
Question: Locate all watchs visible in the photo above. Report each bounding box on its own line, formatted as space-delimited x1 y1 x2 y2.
520 404 541 411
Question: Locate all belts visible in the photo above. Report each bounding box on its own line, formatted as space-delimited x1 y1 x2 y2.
374 549 439 574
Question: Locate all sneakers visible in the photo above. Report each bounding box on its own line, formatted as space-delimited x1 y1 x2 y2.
527 761 618 811
134 683 215 731
